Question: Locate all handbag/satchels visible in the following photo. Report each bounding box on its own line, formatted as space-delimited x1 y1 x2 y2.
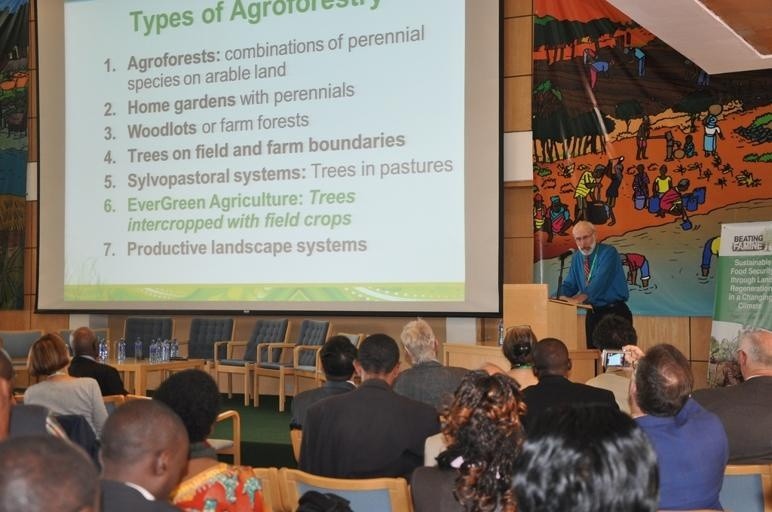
295 490 351 512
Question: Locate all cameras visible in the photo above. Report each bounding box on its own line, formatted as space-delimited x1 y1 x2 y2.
602 350 632 368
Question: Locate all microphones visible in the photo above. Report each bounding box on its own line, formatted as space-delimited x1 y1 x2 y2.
559 248 574 261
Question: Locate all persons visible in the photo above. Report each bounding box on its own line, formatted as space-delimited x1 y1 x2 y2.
67 326 131 396
502 325 539 390
392 318 477 415
410 369 529 510
554 221 637 349
289 336 360 463
622 343 729 510
153 368 264 510
297 333 441 486
511 402 660 510
1 434 102 509
2 349 67 443
585 345 645 416
100 399 188 510
690 329 770 466
23 333 108 440
519 337 620 432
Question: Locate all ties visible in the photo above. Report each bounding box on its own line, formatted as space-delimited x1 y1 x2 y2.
584 256 590 278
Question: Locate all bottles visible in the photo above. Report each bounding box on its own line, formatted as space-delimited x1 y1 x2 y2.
98 338 108 360
497 320 504 344
119 337 126 361
149 337 179 364
135 336 143 362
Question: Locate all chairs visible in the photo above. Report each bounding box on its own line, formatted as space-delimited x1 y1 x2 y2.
0 316 369 417
408 464 520 511
7 388 415 512
713 460 772 512
